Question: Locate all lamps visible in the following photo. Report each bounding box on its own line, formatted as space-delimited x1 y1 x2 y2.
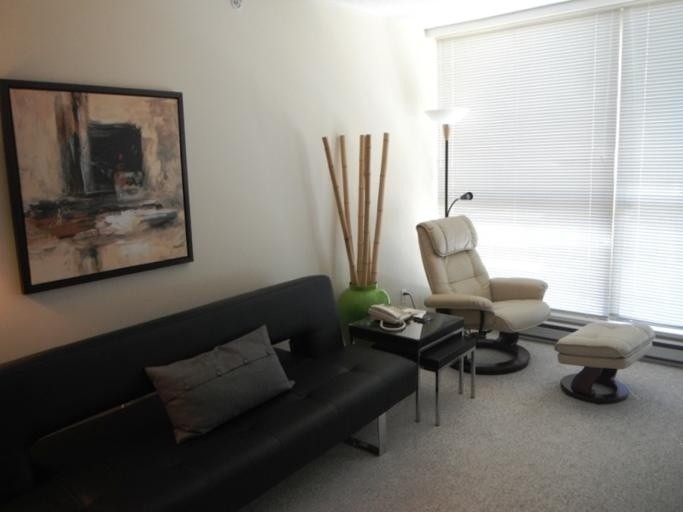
441 122 475 217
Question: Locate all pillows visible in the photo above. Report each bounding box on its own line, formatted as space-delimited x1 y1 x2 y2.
143 322 297 448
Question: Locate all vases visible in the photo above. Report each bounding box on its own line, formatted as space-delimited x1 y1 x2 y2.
334 281 394 346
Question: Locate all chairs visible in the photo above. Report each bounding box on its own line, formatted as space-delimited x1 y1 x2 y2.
415 213 552 377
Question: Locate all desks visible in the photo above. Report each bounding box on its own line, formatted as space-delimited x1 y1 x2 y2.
346 306 477 428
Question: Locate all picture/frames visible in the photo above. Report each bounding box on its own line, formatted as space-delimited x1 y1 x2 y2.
0 79 195 297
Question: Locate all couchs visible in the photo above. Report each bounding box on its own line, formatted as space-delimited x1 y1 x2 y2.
0 273 420 512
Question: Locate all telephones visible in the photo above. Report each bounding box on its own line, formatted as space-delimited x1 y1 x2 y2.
368 303 412 325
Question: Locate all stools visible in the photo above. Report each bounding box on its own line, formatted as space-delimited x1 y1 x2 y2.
553 319 657 407
